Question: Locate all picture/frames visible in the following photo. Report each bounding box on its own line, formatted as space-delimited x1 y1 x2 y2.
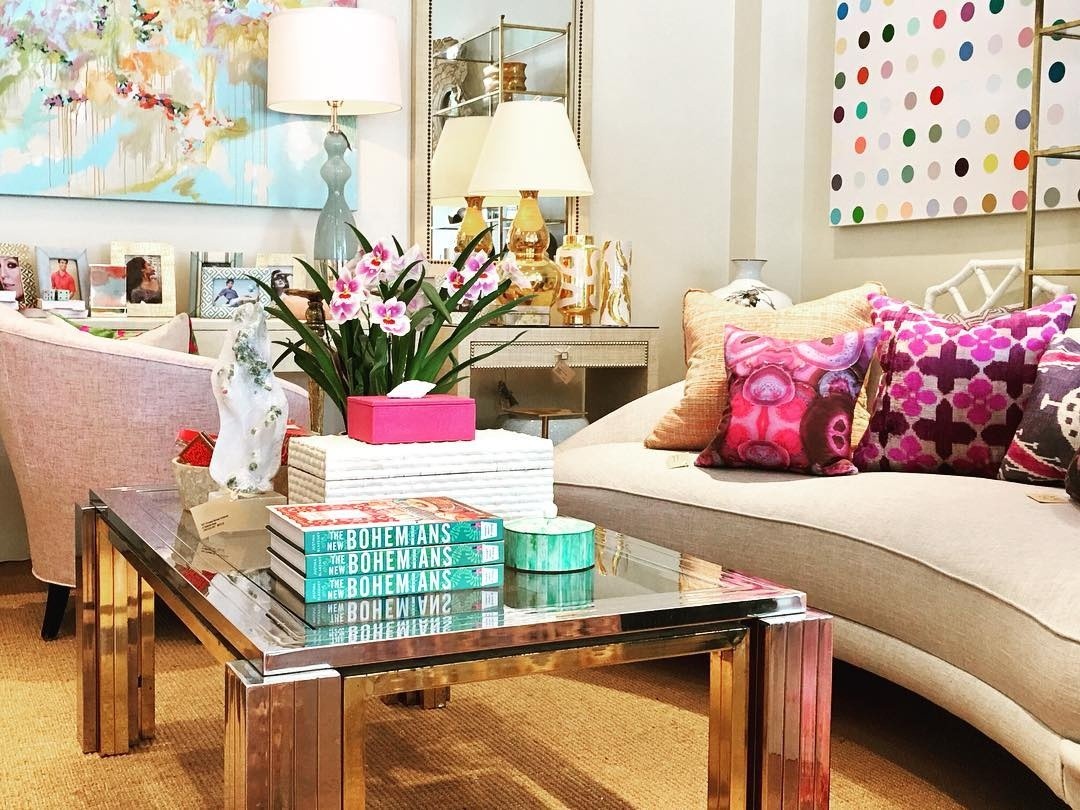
203 253 310 318
0 241 176 317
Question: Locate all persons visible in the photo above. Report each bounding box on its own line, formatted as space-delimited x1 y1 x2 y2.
0 257 24 301
51 259 75 300
126 257 161 303
271 270 293 300
214 279 259 306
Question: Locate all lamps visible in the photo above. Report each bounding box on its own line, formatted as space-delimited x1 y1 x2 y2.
268 10 402 259
433 117 494 251
469 102 588 320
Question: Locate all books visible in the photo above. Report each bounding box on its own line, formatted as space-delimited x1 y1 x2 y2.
267 577 504 648
264 496 505 603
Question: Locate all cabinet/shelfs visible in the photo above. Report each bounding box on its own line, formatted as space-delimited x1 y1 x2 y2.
435 15 574 260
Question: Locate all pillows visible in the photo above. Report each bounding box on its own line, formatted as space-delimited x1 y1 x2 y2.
650 292 1080 500
23 310 198 356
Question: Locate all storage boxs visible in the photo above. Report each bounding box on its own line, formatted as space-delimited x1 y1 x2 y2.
286 428 554 522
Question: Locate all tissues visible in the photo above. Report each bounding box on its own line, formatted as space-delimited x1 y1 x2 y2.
347 380 476 446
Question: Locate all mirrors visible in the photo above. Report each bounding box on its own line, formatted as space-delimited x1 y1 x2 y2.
412 1 591 277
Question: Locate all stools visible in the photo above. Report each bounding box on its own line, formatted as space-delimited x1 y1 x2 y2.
496 406 588 445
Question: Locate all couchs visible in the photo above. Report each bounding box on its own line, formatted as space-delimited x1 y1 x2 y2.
549 377 1080 809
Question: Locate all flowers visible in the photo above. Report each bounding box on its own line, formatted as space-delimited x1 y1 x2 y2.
335 245 496 338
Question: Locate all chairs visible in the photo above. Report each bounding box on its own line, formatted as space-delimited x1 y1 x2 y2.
0 308 311 637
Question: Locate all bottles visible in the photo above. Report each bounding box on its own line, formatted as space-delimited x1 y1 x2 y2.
600 240 634 328
557 235 597 326
711 259 792 312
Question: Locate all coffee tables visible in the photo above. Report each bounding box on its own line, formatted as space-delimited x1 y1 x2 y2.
74 489 833 810
